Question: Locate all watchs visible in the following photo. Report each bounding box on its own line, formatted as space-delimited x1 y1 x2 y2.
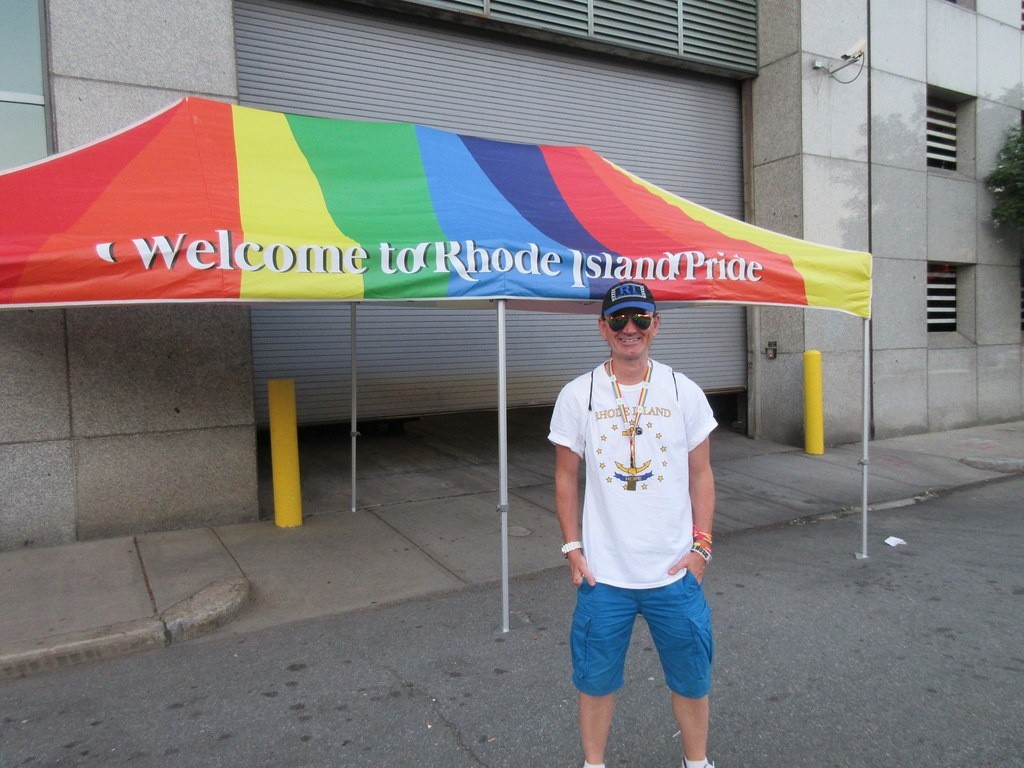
560 541 582 558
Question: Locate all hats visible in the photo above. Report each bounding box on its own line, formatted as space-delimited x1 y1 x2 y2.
601 280 656 319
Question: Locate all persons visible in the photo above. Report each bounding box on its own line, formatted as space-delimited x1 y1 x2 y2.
547 282 721 768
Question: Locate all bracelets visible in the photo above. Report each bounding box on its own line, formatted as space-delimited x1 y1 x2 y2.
691 545 712 564
692 525 713 553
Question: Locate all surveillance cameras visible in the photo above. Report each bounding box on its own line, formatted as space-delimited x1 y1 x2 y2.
841 39 865 61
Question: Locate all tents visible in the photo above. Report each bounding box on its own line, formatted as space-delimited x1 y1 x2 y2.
0 96 876 634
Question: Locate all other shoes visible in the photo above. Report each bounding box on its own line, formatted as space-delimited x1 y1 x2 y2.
680 758 716 768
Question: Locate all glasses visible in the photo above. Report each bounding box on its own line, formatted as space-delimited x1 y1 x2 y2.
604 314 657 332
